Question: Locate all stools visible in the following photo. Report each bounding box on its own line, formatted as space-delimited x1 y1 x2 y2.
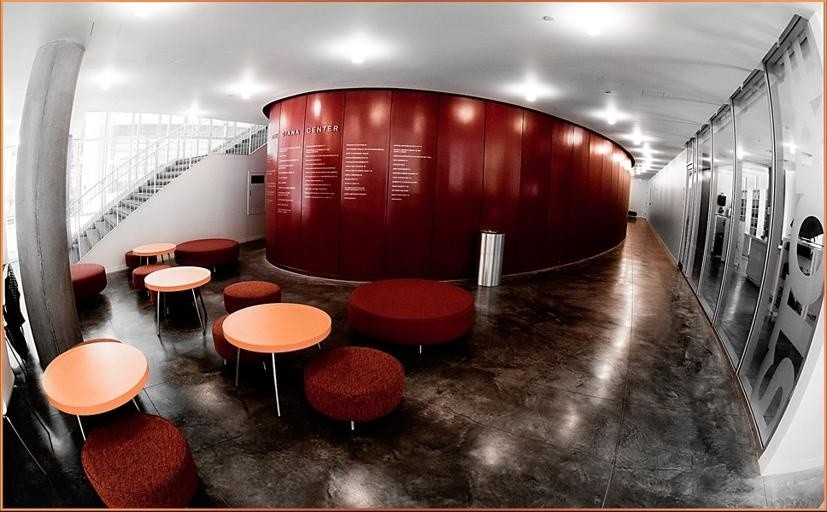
69 239 477 508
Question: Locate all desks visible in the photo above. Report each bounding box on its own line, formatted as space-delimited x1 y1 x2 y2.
43 342 148 441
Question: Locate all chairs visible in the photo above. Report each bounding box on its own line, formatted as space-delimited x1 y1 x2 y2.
4 342 51 477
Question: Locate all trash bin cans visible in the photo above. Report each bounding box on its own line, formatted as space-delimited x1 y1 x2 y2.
477 229 506 287
